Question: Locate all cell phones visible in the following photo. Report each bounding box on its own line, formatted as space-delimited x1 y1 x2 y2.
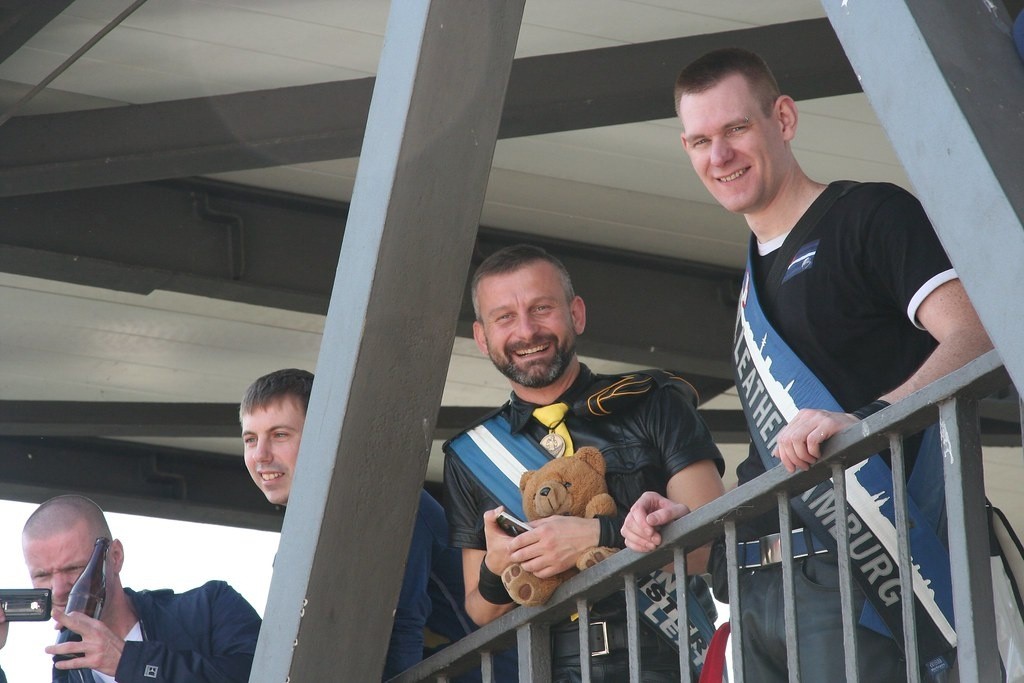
498 511 536 537
0 588 52 621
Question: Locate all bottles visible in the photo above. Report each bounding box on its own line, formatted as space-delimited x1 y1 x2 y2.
51 537 111 671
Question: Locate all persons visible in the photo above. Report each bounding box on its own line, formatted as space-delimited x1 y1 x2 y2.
622 49 998 683
240 367 521 683
0 495 263 683
442 243 726 683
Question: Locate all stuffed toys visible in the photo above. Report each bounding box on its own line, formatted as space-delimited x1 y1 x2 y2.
500 446 624 608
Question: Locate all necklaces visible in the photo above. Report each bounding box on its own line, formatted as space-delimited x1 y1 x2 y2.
509 406 574 458
